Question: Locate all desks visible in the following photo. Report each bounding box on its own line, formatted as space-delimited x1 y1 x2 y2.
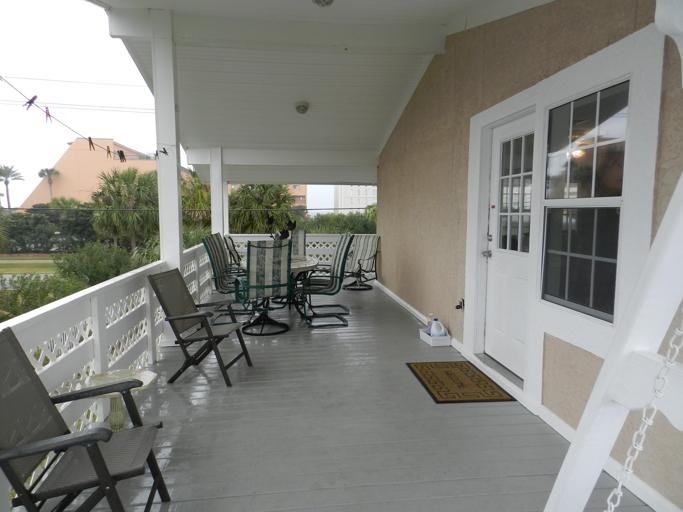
239 254 319 319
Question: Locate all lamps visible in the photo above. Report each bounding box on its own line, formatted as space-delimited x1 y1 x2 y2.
293 102 309 114
311 0 334 9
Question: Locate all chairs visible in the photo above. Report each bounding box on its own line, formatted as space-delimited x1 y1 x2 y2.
200 231 253 325
0 326 172 511
146 267 252 386
235 238 294 336
294 232 354 328
223 232 380 317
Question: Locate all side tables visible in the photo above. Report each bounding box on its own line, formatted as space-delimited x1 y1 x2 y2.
87 367 159 432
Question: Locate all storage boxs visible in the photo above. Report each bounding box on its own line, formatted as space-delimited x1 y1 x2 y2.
419 326 451 346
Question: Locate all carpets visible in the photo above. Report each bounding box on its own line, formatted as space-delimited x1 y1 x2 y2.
405 361 515 402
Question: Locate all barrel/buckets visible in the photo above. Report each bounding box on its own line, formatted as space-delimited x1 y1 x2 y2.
430 318 445 336
